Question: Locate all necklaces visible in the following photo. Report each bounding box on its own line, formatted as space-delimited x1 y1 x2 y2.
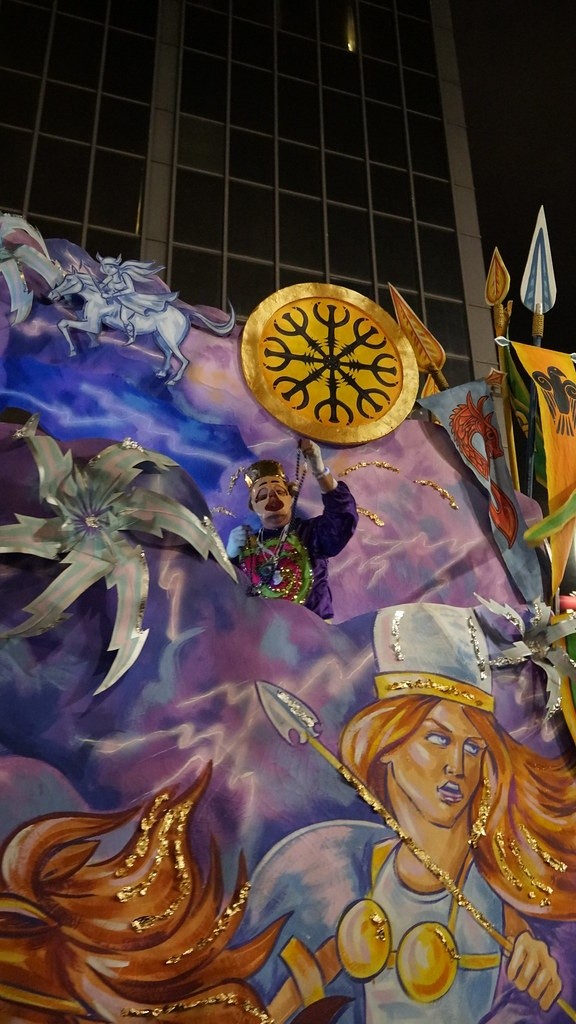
245 439 315 605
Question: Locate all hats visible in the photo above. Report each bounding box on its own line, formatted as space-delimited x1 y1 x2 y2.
244 460 288 489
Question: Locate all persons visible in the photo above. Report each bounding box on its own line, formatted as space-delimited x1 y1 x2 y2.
227 438 360 625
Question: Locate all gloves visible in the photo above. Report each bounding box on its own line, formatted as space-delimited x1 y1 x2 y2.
225 525 253 559
302 438 325 475
